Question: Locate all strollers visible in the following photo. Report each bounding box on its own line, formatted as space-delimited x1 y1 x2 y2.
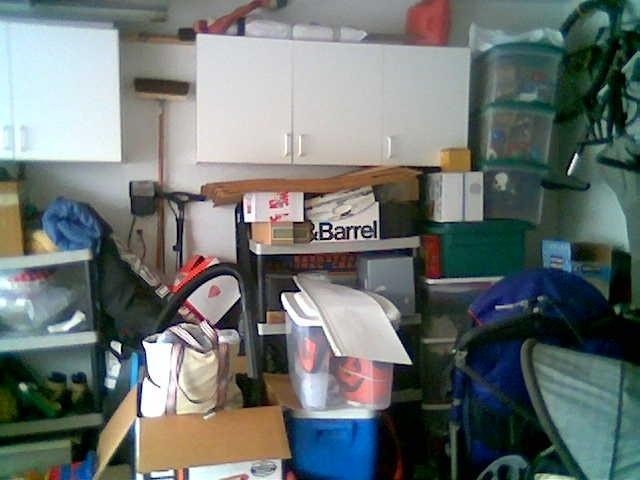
453 312 640 480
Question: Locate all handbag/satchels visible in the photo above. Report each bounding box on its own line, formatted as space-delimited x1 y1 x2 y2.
140 324 244 417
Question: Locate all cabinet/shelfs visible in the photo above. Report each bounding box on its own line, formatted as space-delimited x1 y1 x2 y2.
247 233 423 403
0 248 106 437
1 21 123 164
194 31 381 168
379 43 472 166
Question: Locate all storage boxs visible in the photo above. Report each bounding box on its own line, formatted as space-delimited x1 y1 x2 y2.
464 172 484 222
421 220 536 279
471 162 549 223
471 103 556 164
280 286 401 480
425 173 463 222
91 371 306 480
471 43 563 104
441 149 471 173
420 403 459 480
421 337 457 404
421 277 504 338
